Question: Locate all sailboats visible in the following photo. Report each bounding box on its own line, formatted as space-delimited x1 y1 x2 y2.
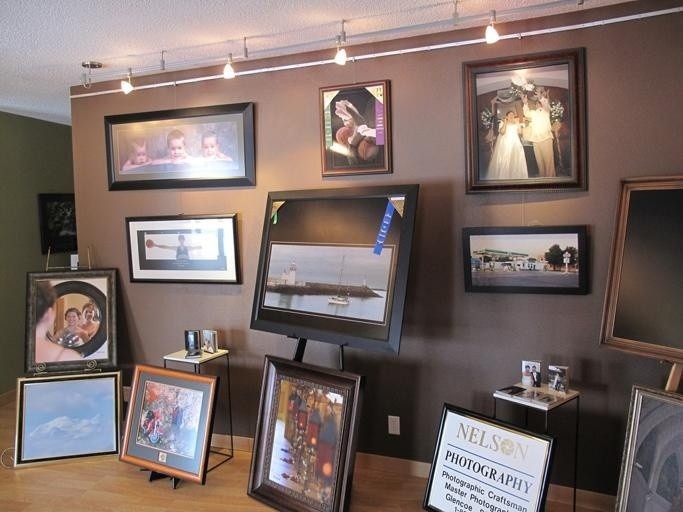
328 255 352 305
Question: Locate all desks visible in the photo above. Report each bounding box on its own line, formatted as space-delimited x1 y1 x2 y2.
163 347 233 474
493 382 580 512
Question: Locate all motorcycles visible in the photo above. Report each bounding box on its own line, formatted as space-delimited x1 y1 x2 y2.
141 409 163 445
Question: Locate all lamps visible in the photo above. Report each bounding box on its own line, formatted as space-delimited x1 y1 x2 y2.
334 48 347 65
120 79 132 94
485 24 498 44
223 64 235 79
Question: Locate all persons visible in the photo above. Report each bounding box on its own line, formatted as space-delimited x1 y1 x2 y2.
531 365 541 387
151 235 204 260
76 301 101 336
34 280 83 361
189 131 231 162
519 89 557 177
150 130 193 166
49 303 88 345
121 136 153 173
522 364 533 386
481 111 529 181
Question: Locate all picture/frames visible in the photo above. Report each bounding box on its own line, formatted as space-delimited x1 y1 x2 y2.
317 78 393 178
462 48 588 195
246 354 365 512
103 100 256 192
423 402 558 512
599 175 683 365
462 225 590 295
615 384 683 512
119 364 220 485
248 183 419 357
37 193 78 254
22 266 121 379
12 367 124 471
125 214 242 285
